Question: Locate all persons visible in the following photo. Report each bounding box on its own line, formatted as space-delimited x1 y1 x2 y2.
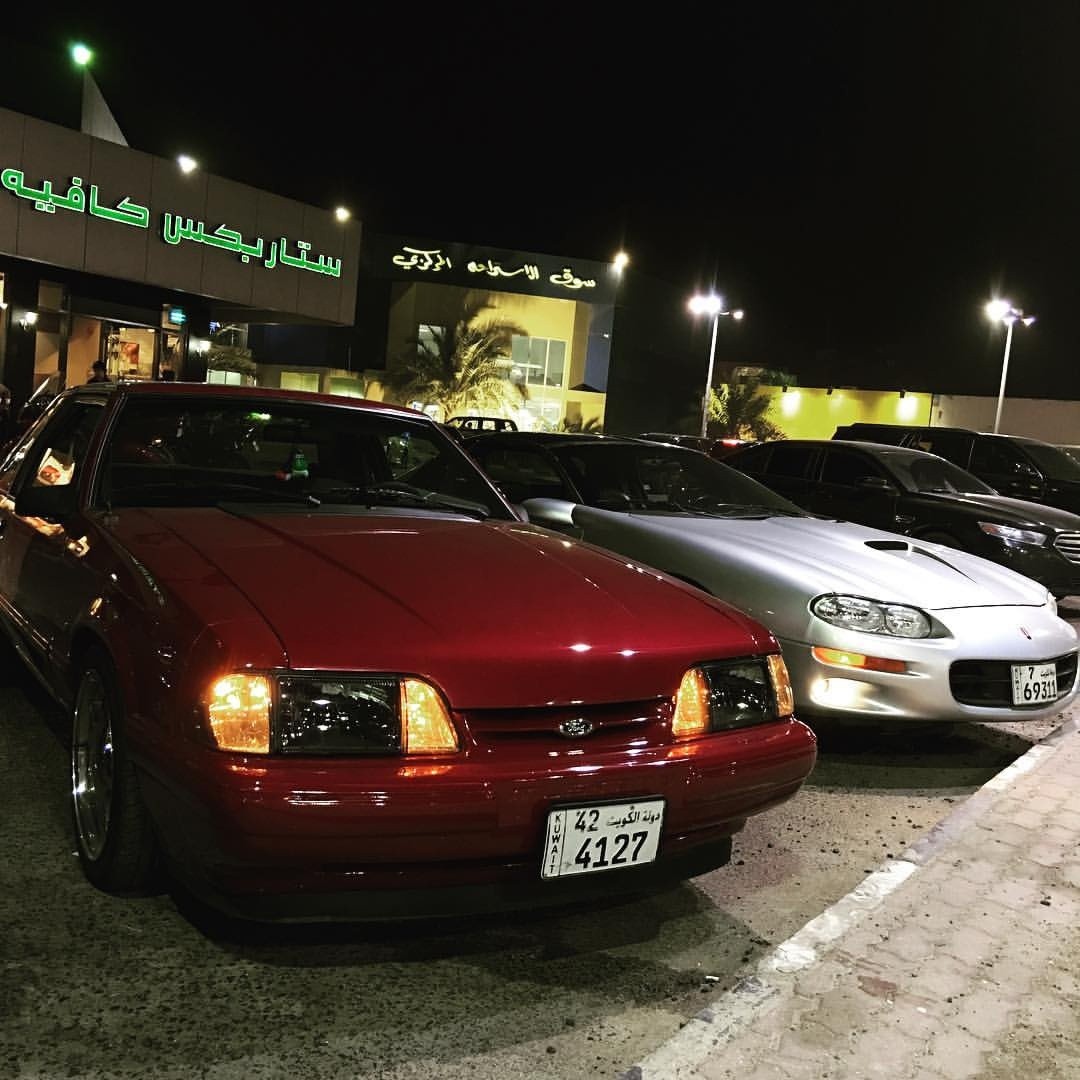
85 359 112 383
160 368 176 382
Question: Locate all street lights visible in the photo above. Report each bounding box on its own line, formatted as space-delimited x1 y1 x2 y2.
684 295 747 437
983 299 1036 435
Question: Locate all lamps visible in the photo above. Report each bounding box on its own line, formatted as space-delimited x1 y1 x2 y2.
827 388 833 395
783 385 787 392
20 312 39 338
900 391 906 398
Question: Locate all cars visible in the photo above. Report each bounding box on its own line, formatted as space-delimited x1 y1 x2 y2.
1 380 818 938
433 418 1079 742
669 440 1080 606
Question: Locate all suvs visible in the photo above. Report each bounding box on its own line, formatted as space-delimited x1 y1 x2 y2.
830 420 1078 519
